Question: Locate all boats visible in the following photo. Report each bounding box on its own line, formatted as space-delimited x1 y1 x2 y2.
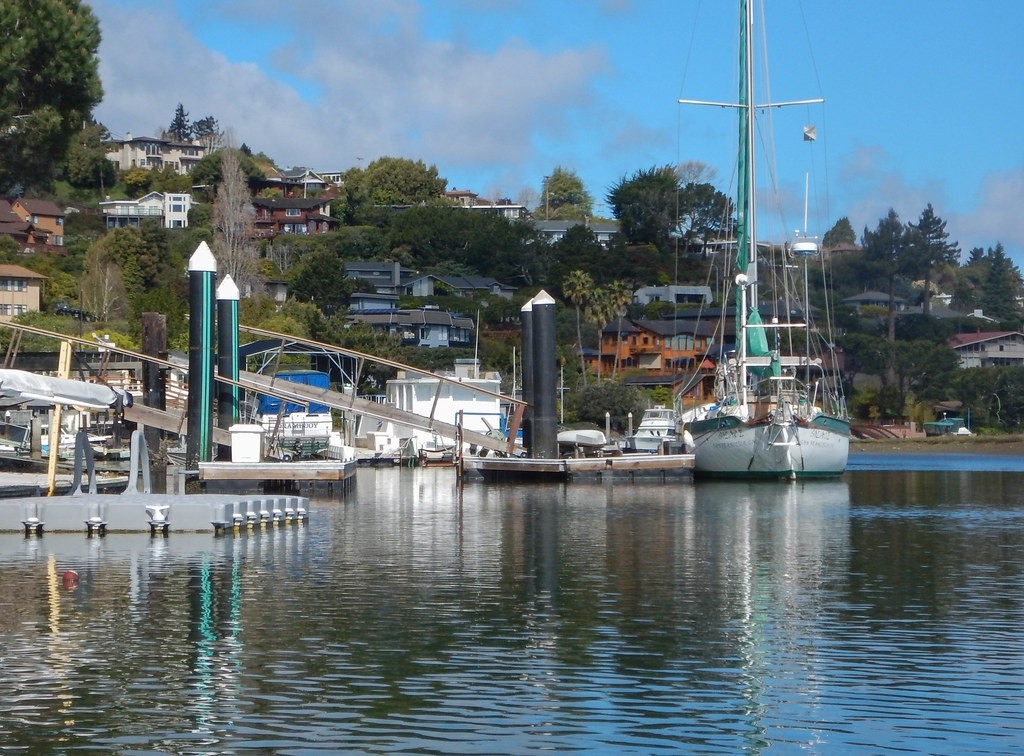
452 402 694 475
615 403 685 455
0 339 361 496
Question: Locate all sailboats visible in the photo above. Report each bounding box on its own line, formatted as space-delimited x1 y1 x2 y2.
675 0 851 477
